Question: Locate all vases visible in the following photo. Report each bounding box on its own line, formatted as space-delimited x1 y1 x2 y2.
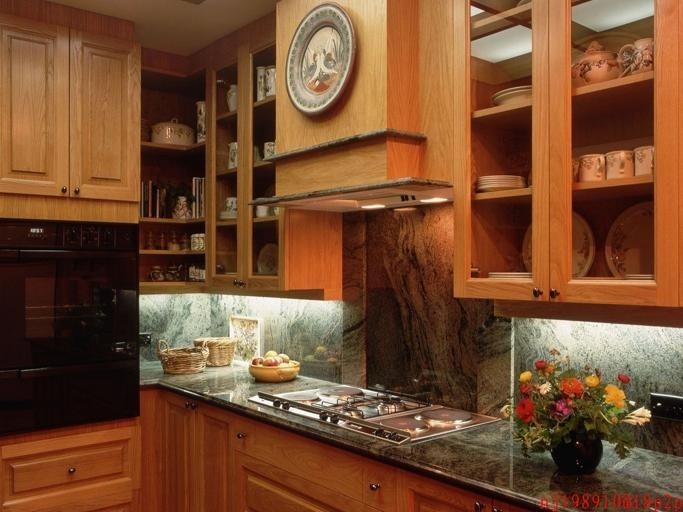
551 418 603 475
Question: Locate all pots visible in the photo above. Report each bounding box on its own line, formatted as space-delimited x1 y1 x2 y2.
151 118 195 146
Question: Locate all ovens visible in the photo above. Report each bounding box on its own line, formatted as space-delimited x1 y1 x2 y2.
0 219 142 437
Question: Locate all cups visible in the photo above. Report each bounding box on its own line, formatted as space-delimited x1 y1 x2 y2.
617 37 655 78
225 141 279 220
572 146 653 183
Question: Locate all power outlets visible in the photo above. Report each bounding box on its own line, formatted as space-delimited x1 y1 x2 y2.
649 393 683 422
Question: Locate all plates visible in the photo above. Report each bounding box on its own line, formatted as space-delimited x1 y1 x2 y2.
488 201 655 279
490 85 532 105
285 3 356 117
229 316 260 366
257 243 278 275
219 212 238 220
477 176 524 192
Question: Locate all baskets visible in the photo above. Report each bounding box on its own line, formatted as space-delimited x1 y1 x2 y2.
195 338 235 366
158 340 208 374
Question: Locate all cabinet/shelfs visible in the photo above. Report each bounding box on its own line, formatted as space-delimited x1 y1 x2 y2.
454 0 683 304
0 420 143 512
208 39 343 303
1 0 140 222
402 468 536 512
235 414 402 512
155 385 235 512
139 66 210 287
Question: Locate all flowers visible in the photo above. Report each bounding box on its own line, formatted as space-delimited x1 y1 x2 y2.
500 348 651 460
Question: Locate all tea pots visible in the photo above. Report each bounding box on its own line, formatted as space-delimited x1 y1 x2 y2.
150 264 183 281
571 40 639 86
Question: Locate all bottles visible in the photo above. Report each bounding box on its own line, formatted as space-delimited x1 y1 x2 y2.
255 65 276 102
183 264 206 282
145 231 207 252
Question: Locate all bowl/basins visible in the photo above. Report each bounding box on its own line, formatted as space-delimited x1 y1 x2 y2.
249 360 300 383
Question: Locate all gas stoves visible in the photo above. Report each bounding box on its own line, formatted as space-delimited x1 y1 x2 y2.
246 384 501 445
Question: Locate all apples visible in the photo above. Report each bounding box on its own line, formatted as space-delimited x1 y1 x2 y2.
314 346 328 360
252 357 264 365
278 354 289 363
264 351 278 358
263 358 278 367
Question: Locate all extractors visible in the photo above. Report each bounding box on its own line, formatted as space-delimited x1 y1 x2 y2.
247 176 453 213
